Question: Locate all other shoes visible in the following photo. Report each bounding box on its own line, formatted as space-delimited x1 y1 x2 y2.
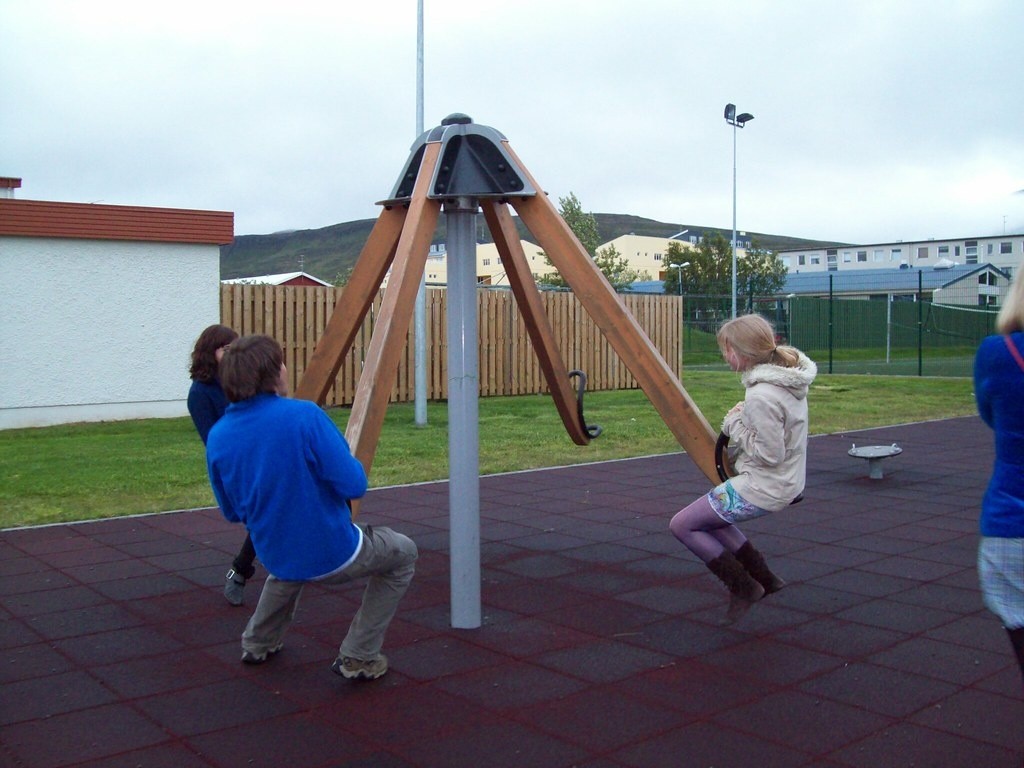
224 567 245 605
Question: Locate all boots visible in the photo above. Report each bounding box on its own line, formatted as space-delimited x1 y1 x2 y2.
734 540 785 598
705 548 765 626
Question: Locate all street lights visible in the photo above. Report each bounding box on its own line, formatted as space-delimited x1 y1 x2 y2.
670 262 690 296
725 103 755 318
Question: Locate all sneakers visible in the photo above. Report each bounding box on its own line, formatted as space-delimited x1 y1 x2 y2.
240 647 280 665
330 652 387 678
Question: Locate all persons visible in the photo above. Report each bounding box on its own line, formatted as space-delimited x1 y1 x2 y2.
205 335 418 682
186 324 257 604
670 315 818 627
974 262 1024 671
